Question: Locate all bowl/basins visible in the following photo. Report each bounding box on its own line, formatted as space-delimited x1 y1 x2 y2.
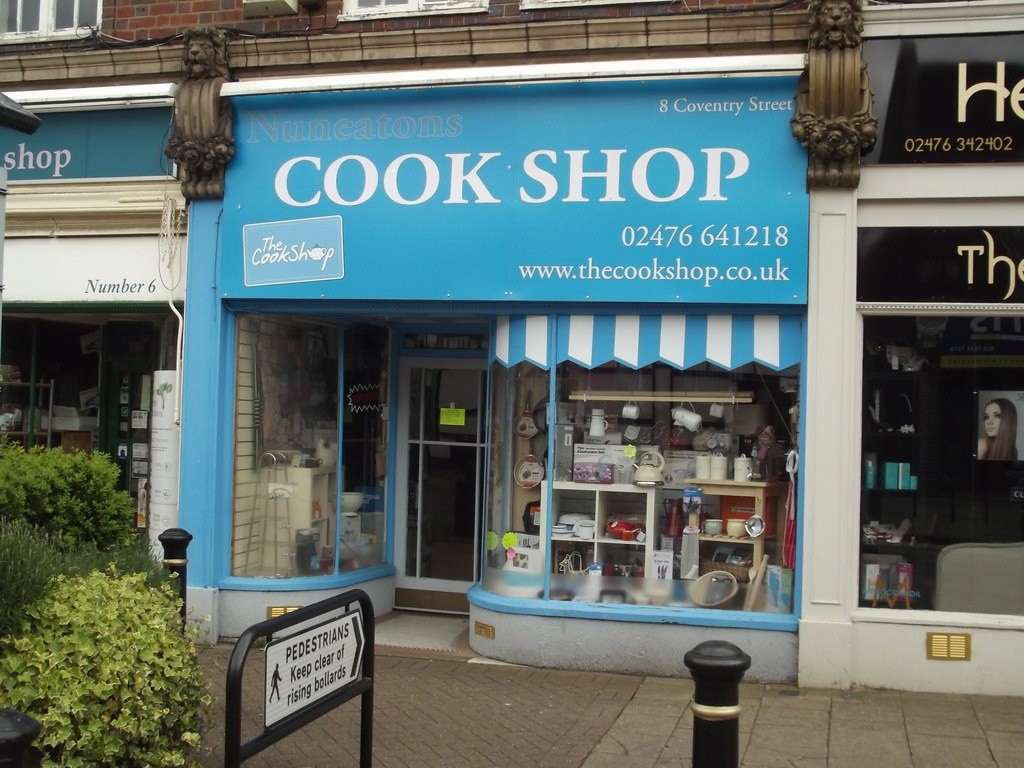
557 511 593 525
341 492 363 516
554 532 574 538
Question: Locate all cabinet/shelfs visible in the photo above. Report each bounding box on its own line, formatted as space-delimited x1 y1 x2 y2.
259 465 345 577
859 369 930 607
0 377 54 453
539 480 660 578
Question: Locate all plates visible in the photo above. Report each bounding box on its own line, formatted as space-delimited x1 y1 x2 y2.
746 516 765 536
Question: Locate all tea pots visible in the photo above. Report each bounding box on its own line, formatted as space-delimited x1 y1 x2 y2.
604 520 634 540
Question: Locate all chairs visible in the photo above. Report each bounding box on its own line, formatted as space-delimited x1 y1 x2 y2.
935 542 1024 615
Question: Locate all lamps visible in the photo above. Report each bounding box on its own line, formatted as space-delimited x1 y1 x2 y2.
569 369 754 404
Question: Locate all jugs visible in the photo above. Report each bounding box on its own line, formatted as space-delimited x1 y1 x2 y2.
671 400 702 432
664 498 684 542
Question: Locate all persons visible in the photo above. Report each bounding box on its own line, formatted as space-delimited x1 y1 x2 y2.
978 398 1019 461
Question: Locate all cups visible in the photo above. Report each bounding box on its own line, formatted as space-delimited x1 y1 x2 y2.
622 400 639 419
580 525 593 539
552 526 567 539
588 415 608 436
622 530 632 542
710 404 725 417
727 518 747 537
702 519 722 535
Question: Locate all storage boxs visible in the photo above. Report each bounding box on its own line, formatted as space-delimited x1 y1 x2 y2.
653 550 674 580
663 449 707 481
721 495 776 535
636 445 661 467
41 416 97 432
895 562 913 601
898 462 911 489
328 515 360 561
584 431 622 445
861 562 879 600
545 402 585 425
555 424 581 481
660 535 673 550
881 461 898 489
573 444 636 483
766 565 793 614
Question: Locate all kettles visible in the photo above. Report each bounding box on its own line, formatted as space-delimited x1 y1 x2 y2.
634 452 664 482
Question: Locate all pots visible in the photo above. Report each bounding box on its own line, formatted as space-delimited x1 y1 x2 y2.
513 437 544 489
514 390 538 439
690 571 739 607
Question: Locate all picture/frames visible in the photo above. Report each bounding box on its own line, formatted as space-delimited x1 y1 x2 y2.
253 342 314 471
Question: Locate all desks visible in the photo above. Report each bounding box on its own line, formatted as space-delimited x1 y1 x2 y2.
685 477 771 584
